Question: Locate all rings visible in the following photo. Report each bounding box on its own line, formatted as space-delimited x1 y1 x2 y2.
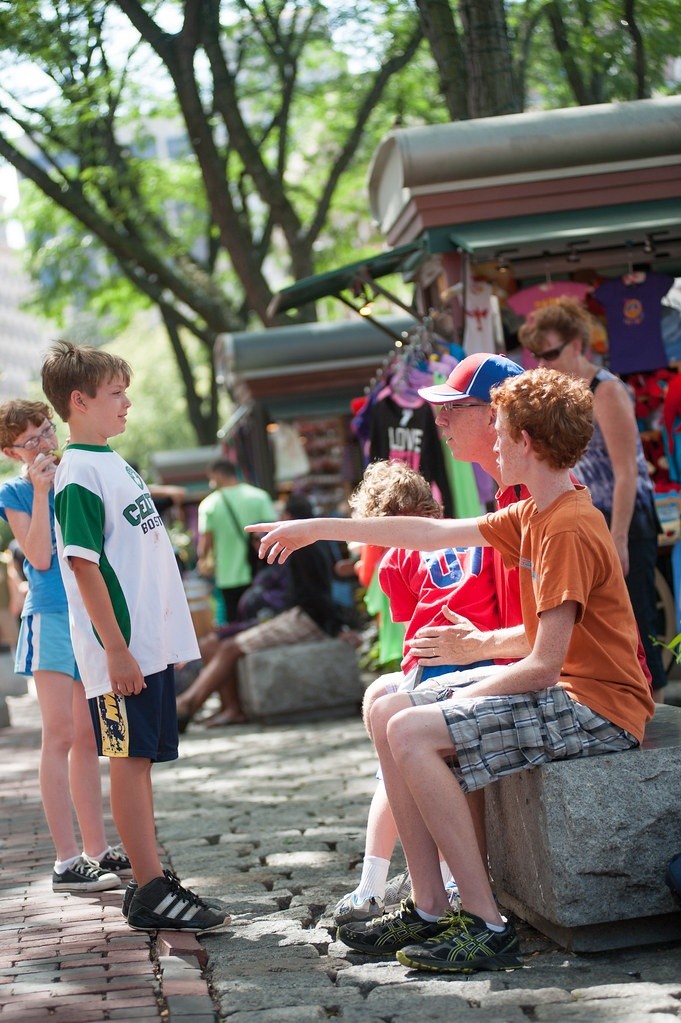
432 648 435 656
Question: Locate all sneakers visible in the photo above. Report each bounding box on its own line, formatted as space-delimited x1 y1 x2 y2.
86 849 164 880
339 903 462 956
52 858 121 892
383 868 417 912
433 881 500 914
333 891 385 928
395 912 524 973
122 880 231 931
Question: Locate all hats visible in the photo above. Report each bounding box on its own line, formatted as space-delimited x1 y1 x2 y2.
417 352 526 405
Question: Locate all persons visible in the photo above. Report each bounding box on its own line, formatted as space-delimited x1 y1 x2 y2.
201 524 298 732
243 370 656 978
0 400 136 892
359 352 586 913
38 338 230 929
197 463 280 634
333 461 495 918
172 495 337 729
518 294 669 702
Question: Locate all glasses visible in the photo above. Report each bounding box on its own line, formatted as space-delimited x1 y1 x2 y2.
11 423 57 451
532 340 572 361
443 402 489 411
